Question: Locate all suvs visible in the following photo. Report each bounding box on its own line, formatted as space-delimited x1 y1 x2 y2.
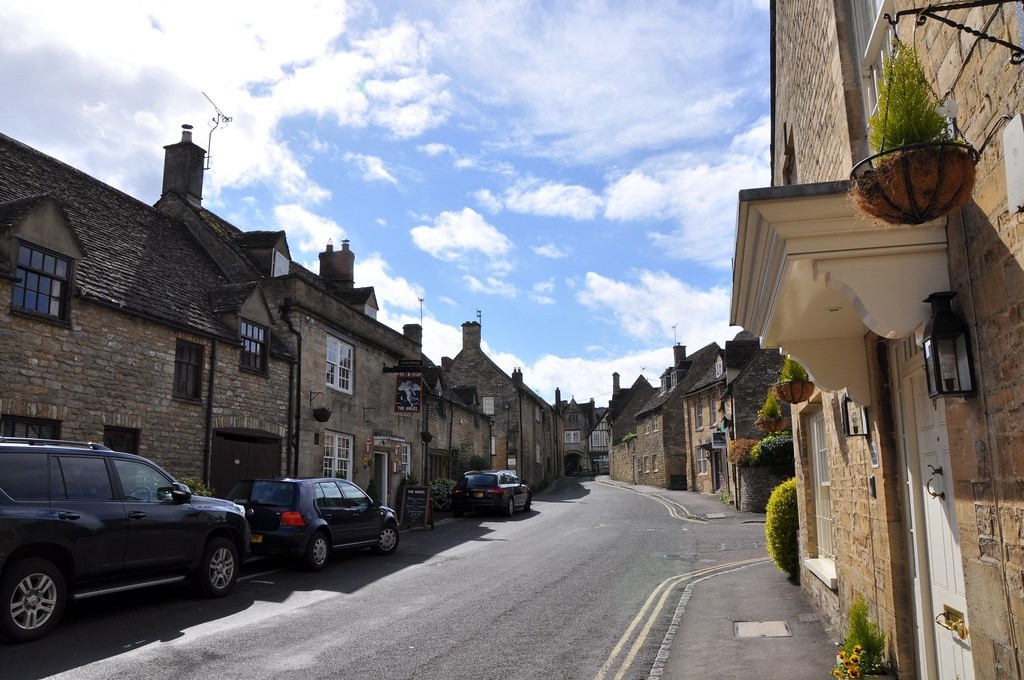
0 437 254 647
450 470 533 520
222 475 402 574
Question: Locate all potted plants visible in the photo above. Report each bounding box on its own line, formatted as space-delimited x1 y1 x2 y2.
772 355 815 404
848 35 981 227
313 407 333 422
839 590 897 680
754 387 789 432
420 431 435 443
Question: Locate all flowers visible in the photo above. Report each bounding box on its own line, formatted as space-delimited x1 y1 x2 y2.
832 644 862 680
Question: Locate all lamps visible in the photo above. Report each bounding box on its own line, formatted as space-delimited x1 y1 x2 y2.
366 436 373 454
921 291 977 400
394 443 402 456
841 393 870 437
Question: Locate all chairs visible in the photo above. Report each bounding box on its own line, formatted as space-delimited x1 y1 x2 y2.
80 463 112 499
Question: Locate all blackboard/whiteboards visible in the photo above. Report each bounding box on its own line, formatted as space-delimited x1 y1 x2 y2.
400 485 434 527
